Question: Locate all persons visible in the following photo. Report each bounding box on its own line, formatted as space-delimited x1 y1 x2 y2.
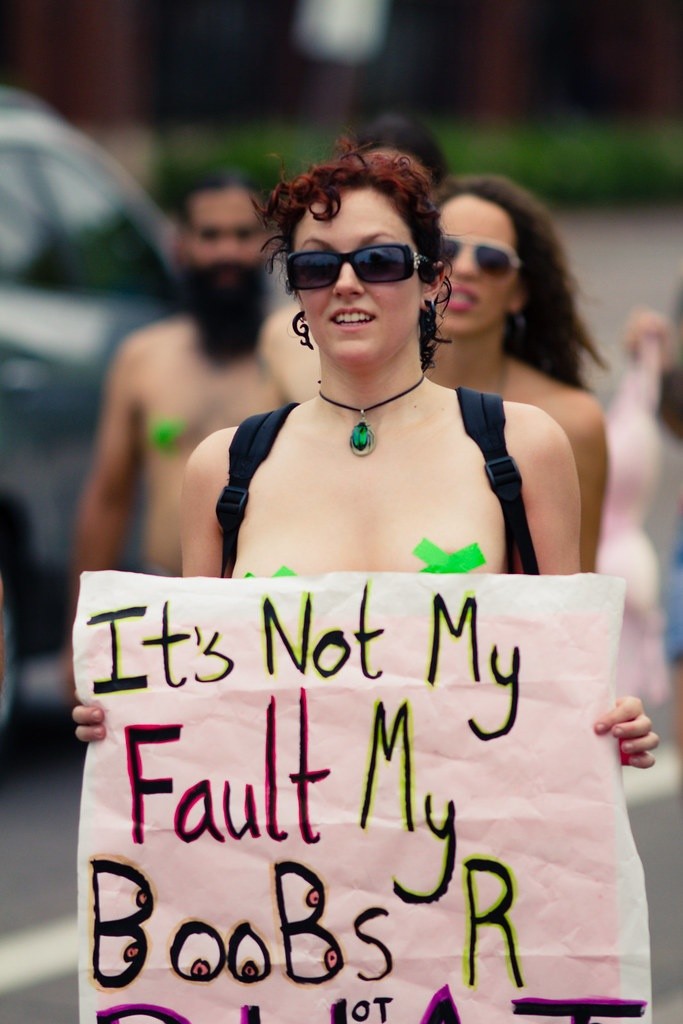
625 313 683 657
259 124 447 403
64 156 283 699
72 145 660 768
422 171 606 575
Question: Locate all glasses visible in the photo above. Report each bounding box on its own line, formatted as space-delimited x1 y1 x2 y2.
441 237 523 275
285 243 428 290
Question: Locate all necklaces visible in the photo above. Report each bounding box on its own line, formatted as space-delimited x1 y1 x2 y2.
319 374 424 456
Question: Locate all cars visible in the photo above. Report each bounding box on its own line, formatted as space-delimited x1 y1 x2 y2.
0 82 294 796
